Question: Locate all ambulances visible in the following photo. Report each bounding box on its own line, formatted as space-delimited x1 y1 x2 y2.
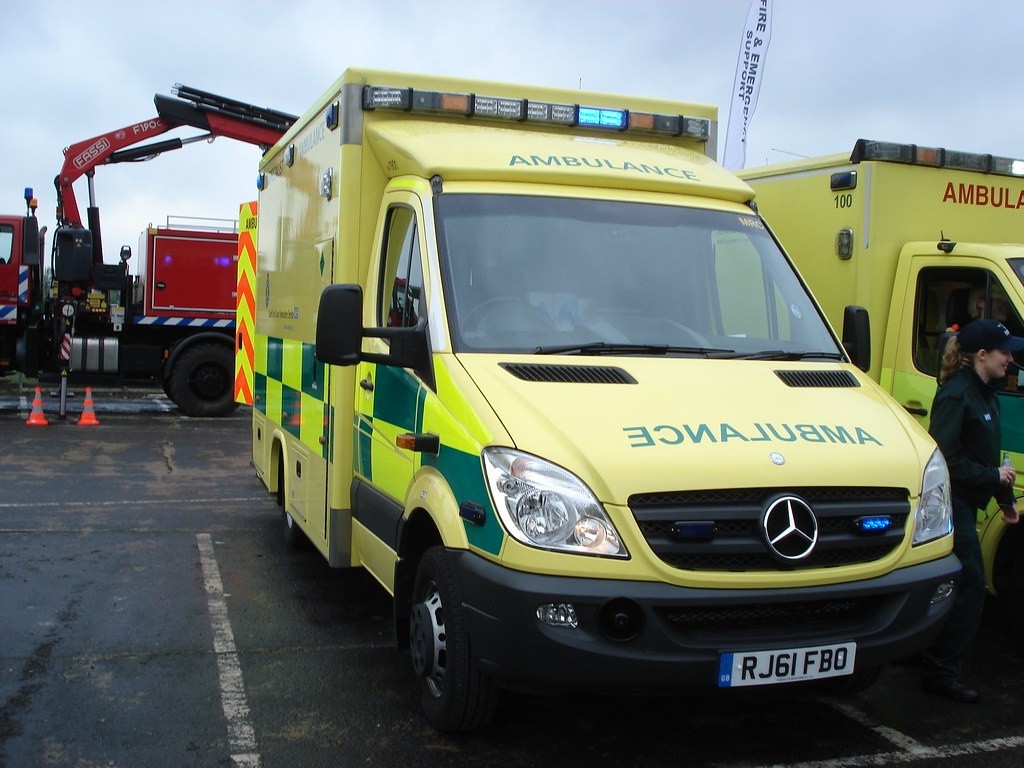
247 66 960 745
695 137 1023 647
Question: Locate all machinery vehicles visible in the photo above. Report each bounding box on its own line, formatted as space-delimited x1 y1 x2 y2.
0 83 306 419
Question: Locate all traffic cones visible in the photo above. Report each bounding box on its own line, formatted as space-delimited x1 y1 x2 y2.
25 388 50 427
71 385 99 427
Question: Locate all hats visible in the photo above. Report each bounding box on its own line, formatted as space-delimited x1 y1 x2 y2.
956 319 1024 351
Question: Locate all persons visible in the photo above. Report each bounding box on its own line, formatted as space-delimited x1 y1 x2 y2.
967 272 1008 321
923 320 1024 702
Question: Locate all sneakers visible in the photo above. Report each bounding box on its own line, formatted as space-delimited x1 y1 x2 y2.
905 647 947 668
923 669 977 703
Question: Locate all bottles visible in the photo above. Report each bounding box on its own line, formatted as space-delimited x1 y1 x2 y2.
999 453 1015 510
